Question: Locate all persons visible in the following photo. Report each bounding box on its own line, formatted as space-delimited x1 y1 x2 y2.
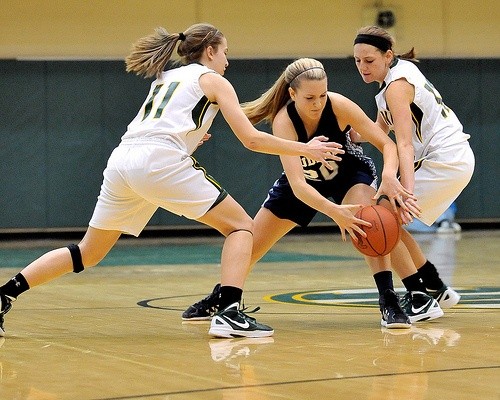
0 24 344 339
349 25 475 326
180 58 417 329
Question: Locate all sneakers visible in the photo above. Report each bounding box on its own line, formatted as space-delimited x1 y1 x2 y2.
0 295 17 336
378 289 412 328
208 300 274 338
181 283 221 321
399 291 444 323
426 284 461 309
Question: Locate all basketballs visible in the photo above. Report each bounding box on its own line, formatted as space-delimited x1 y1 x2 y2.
350 205 401 257
